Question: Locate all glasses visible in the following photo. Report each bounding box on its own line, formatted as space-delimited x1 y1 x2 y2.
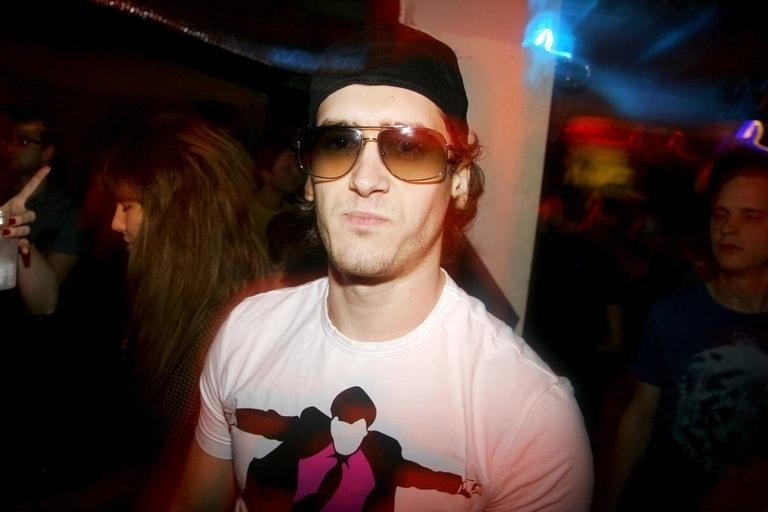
297 125 460 181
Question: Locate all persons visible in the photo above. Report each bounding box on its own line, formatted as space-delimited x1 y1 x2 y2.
525 136 768 510
171 21 595 511
0 104 325 511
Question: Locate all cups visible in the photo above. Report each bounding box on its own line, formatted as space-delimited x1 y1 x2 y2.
0 210 20 293
